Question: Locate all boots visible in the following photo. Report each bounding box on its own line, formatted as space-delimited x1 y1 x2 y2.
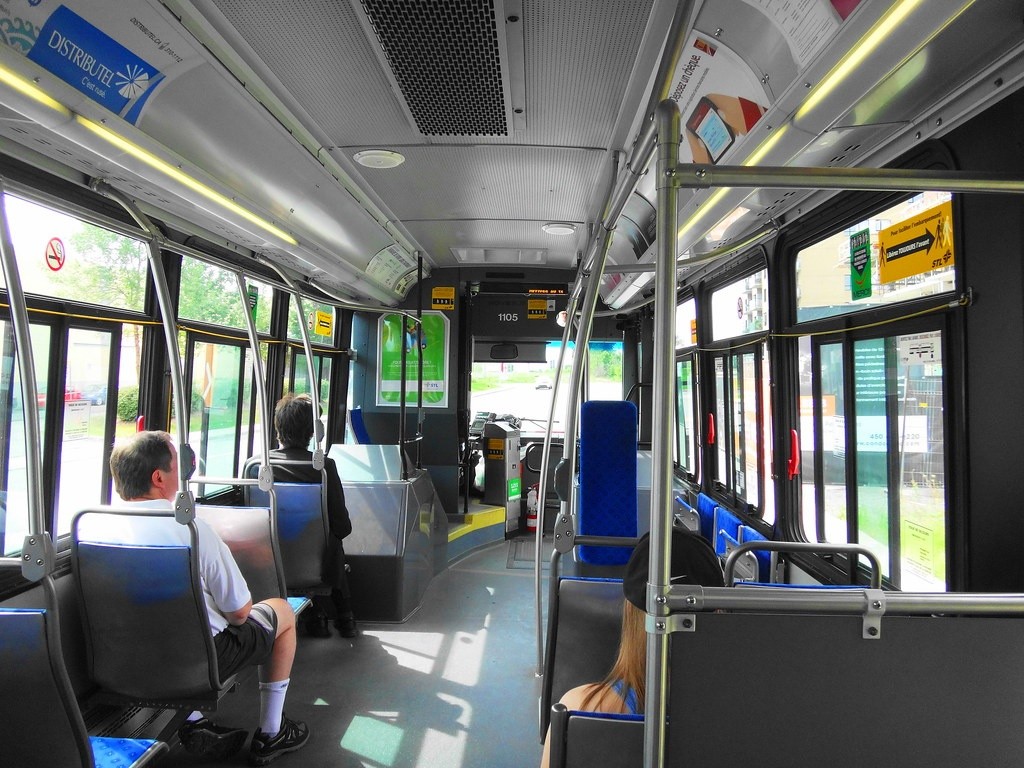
330 571 359 636
305 596 330 637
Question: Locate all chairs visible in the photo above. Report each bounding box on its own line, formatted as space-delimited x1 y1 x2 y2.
0 448 882 768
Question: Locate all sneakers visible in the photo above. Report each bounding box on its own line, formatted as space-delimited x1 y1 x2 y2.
250 713 311 765
178 717 249 761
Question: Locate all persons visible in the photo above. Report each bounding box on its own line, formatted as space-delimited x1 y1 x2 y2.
108 430 310 763
249 392 361 638
540 526 724 768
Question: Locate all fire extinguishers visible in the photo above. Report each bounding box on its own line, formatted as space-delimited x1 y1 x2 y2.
527 483 539 533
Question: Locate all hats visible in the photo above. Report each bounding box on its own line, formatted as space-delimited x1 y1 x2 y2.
623 526 724 612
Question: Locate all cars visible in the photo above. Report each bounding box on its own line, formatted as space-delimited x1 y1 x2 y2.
31 384 82 410
84 385 108 406
534 376 552 390
